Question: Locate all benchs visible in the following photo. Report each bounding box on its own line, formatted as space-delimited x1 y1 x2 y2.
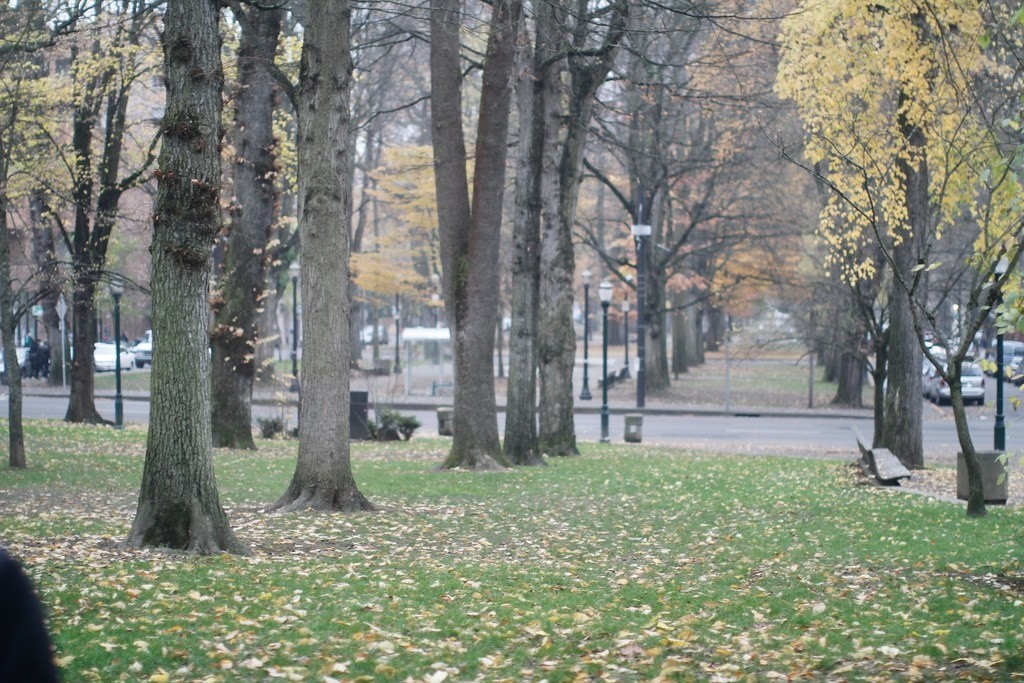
849 423 913 484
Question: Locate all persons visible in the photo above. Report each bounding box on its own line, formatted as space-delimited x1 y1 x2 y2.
29 339 49 378
25 331 32 347
974 331 986 358
0 548 68 683
925 333 939 344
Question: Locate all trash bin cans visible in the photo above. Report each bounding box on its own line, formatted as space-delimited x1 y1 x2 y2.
380 358 391 375
349 391 368 439
437 408 454 436
624 414 643 443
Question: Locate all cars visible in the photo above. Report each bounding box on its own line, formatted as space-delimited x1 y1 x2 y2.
359 324 389 345
133 328 152 369
93 342 135 372
923 330 1024 407
0 346 36 380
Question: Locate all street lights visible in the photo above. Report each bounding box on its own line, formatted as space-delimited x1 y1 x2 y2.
992 251 1008 453
108 274 125 428
392 312 403 374
621 299 632 380
578 270 594 401
597 279 613 443
288 262 301 393
